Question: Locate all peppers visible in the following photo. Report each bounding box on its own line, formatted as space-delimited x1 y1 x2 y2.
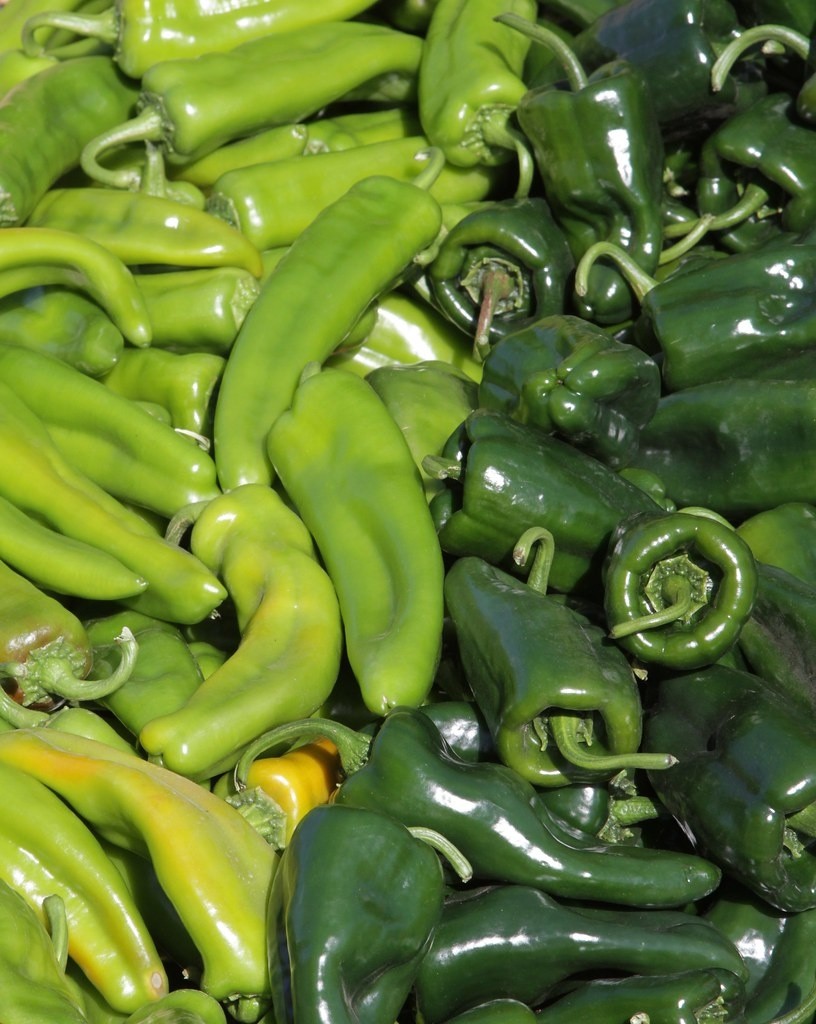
0 0 816 1024
